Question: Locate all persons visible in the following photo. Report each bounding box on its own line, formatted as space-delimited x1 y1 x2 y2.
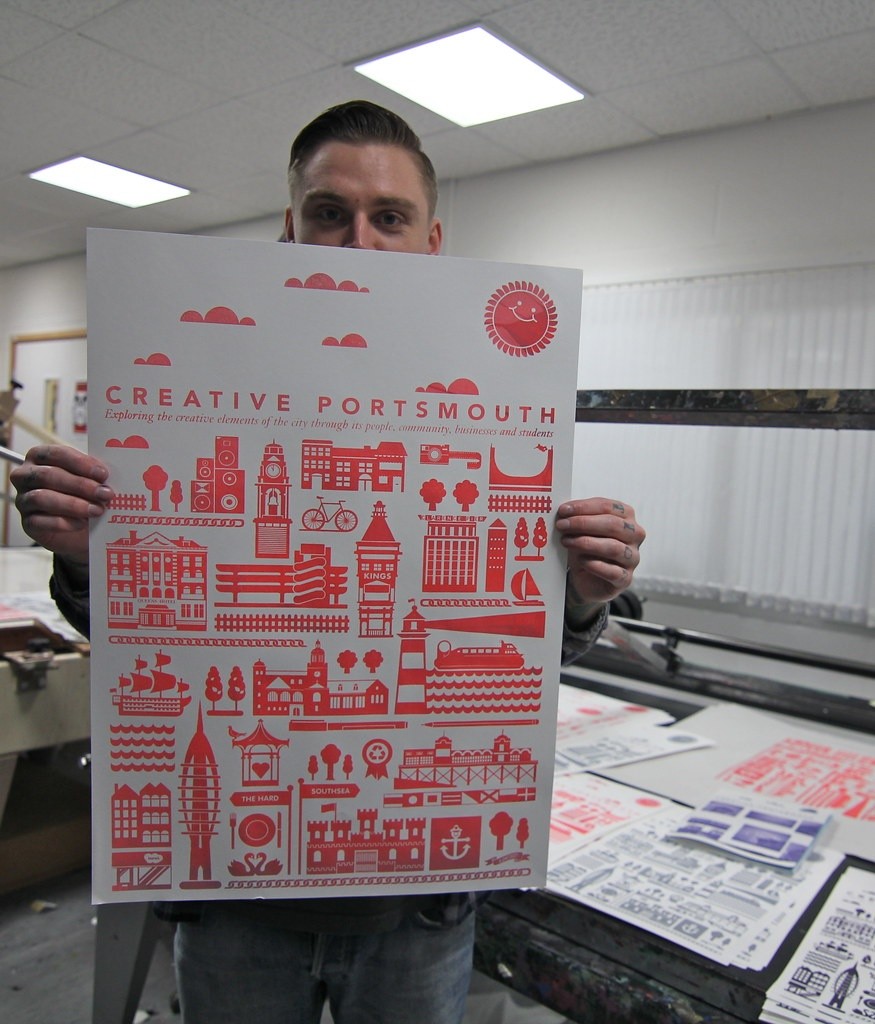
10 100 647 1024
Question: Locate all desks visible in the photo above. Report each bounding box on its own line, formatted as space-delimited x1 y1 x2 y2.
0 649 92 815
